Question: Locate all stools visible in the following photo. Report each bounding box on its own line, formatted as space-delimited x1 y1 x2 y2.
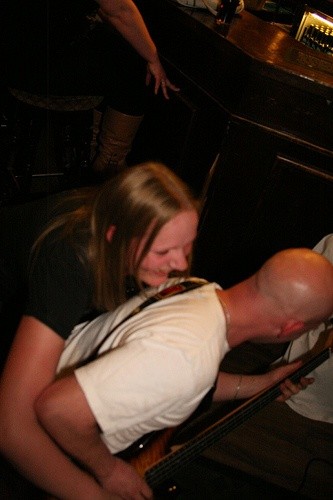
6 88 102 194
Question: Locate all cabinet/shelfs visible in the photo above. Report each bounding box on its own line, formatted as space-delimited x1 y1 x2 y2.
289 4 333 57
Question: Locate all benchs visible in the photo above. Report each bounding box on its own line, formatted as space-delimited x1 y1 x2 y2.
188 356 333 499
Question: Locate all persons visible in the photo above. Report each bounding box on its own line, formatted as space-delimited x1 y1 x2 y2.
257 225 332 468
0 1 183 178
34 246 333 500
0 164 203 500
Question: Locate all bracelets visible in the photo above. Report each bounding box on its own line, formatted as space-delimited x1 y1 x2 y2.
233 373 245 402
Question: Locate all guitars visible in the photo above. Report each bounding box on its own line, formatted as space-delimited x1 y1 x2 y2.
118 328 333 500
